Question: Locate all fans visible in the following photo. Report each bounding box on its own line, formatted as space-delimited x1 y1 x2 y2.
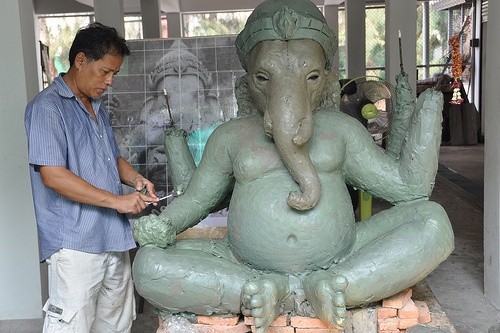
340 75 398 222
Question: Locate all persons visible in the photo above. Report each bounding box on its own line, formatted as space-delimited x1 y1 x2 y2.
23 21 160 333
